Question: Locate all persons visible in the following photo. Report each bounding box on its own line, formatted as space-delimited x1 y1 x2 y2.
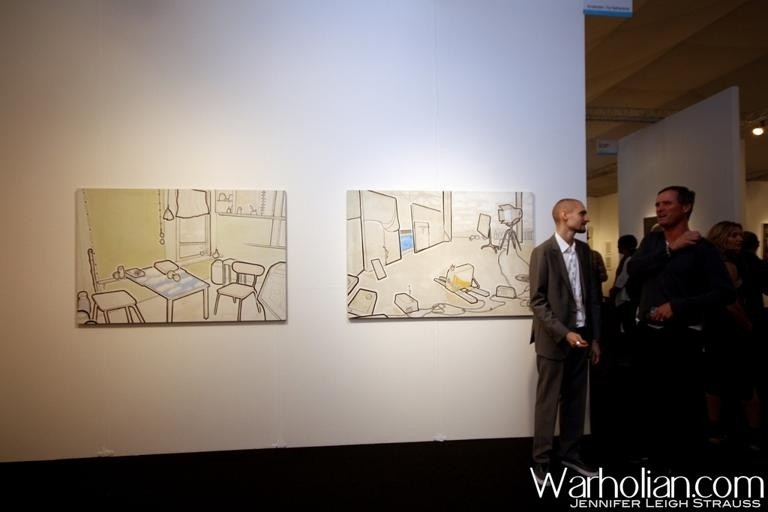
529 199 602 486
588 185 768 481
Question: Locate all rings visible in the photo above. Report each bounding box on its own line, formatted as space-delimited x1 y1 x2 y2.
575 341 580 347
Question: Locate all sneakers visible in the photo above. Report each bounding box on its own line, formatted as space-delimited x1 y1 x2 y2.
533 465 551 487
561 459 599 476
